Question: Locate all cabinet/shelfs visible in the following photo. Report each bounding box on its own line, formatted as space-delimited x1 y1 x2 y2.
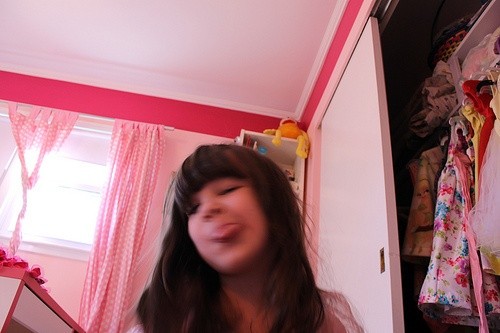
236 128 304 231
235 0 500 333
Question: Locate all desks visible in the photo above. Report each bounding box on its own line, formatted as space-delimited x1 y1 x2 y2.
0 267 87 333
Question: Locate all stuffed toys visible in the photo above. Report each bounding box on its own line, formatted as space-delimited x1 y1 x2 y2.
263 117 310 158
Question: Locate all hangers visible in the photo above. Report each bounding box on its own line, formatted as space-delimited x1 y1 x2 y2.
440 66 500 146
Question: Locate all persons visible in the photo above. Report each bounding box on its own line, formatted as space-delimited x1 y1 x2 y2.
126 143 365 333
